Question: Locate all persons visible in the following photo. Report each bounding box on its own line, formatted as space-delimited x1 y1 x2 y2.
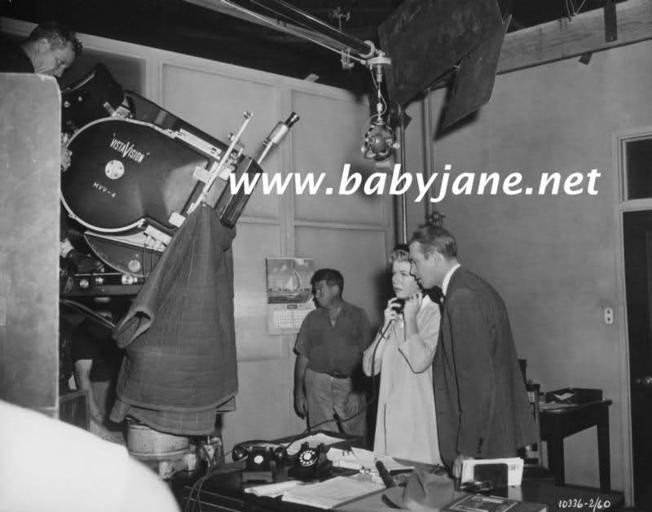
0 18 108 280
361 244 447 470
294 269 376 438
407 224 543 480
70 295 129 446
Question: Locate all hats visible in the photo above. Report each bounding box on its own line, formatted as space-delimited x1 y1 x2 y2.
384 464 456 512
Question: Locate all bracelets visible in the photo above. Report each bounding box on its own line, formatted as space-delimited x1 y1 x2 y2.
379 329 387 339
93 410 103 419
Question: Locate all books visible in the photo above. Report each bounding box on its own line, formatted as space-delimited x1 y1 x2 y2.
440 491 547 512
280 475 387 512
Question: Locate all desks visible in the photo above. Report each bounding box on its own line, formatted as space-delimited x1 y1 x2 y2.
535 398 611 495
169 430 626 511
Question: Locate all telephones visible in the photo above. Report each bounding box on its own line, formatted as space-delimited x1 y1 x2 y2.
231 440 286 483
391 289 429 314
